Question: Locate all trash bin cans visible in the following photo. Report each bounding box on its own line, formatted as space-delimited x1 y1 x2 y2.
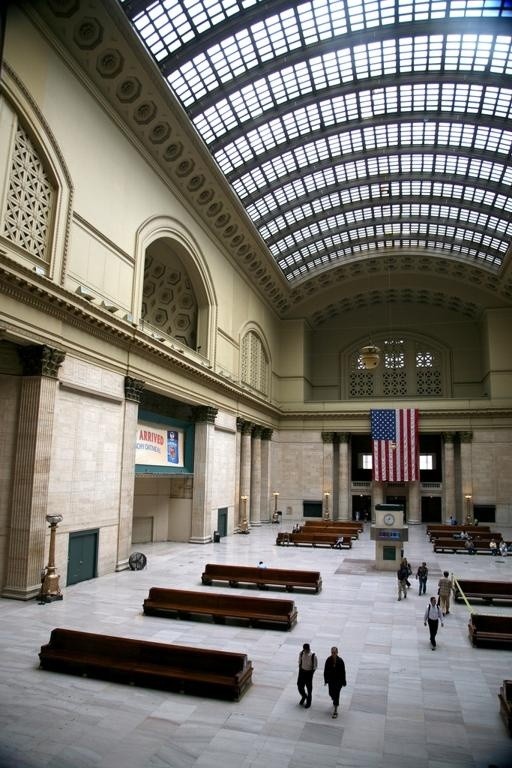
214 531 220 543
277 511 282 522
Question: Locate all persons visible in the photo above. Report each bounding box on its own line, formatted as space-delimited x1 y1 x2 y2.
423 597 444 650
416 562 428 596
294 523 301 532
396 562 409 600
298 642 318 709
332 533 344 550
449 511 512 557
402 555 412 588
323 646 347 719
436 569 452 617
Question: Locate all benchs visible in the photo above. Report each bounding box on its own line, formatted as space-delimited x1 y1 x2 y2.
140 585 299 635
195 562 325 596
467 611 512 651
277 518 511 556
450 575 512 606
493 677 512 737
37 625 256 704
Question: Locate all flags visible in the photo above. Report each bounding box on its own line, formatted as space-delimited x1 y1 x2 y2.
369 407 420 482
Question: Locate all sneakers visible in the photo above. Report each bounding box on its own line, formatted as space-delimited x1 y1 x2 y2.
299 694 307 705
428 610 450 651
305 697 312 708
394 589 428 601
332 710 338 719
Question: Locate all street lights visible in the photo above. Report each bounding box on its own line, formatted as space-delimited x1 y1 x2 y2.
237 494 250 533
323 488 332 522
464 491 474 527
271 489 281 522
39 512 65 602
